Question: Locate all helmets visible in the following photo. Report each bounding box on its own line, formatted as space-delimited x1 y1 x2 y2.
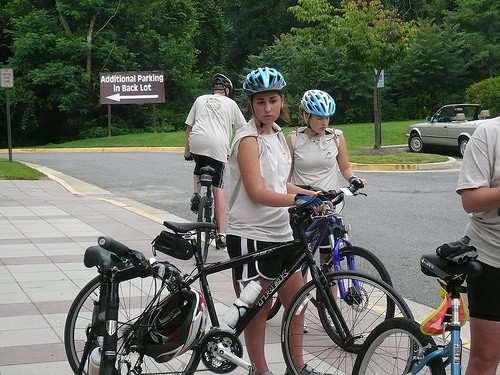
300 89 336 116
242 67 286 94
212 73 234 94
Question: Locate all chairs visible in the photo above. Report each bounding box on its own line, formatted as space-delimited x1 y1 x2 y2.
452 113 467 122
480 110 490 120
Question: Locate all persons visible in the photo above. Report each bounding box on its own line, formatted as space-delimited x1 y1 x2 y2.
456 115 500 375
184 73 250 248
286 89 367 333
224 68 333 375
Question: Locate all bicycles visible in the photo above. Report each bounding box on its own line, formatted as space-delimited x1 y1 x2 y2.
63 220 415 375
83 234 192 375
352 254 482 375
194 167 217 265
297 180 394 353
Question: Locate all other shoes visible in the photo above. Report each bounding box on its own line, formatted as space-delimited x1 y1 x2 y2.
216 233 226 249
191 193 201 212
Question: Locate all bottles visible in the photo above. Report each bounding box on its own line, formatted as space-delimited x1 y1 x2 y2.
89 347 102 375
222 279 262 330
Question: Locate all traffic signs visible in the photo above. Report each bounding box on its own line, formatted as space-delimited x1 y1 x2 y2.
99 69 166 106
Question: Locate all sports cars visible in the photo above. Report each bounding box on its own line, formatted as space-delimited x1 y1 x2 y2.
405 102 494 156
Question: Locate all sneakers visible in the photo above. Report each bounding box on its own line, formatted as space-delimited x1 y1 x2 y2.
283 363 335 375
258 371 274 375
